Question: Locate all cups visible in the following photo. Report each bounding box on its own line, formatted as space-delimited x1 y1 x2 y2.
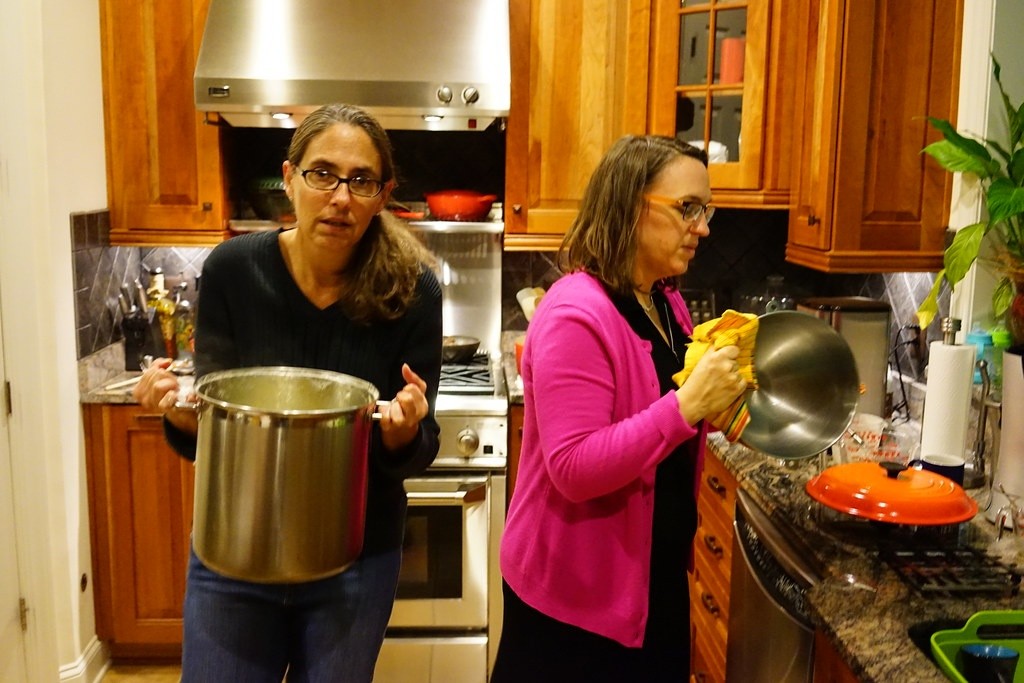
962 643 1019 683
845 413 887 464
720 37 746 84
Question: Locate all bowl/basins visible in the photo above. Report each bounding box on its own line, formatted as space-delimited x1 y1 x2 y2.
442 335 481 362
248 187 294 220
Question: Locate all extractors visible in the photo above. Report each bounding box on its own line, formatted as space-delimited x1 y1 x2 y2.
193 0 512 132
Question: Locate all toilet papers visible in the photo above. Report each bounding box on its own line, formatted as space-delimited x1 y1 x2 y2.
919 339 979 467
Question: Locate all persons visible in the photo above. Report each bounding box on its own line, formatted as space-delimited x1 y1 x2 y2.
135 103 444 683
491 135 747 683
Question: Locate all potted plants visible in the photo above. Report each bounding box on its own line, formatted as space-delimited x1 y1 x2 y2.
905 50 1024 346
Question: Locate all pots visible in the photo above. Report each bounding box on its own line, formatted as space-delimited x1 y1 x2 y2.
424 190 496 222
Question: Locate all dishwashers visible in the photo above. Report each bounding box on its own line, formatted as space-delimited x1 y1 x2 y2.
724 489 822 683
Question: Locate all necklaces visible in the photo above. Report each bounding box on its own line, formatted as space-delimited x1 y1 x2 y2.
643 296 654 311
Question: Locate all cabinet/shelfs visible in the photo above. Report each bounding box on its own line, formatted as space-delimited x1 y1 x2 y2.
688 433 742 683
81 403 197 661
506 0 651 267
94 0 232 249
654 0 788 212
780 0 966 278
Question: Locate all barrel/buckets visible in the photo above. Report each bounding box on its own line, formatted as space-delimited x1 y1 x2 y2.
173 367 393 583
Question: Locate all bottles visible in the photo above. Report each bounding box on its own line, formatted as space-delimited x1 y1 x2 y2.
148 268 175 360
174 297 194 361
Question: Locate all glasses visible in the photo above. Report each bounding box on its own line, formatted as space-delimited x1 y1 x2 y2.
294 166 385 197
642 192 716 223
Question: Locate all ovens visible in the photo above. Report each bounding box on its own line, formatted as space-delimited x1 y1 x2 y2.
370 477 491 682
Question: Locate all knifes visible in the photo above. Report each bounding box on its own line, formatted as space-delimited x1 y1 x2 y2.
117 282 150 318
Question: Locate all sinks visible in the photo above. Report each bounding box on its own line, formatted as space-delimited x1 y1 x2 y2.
906 614 1024 683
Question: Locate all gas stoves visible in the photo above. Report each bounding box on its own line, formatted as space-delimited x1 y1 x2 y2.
425 351 509 472
